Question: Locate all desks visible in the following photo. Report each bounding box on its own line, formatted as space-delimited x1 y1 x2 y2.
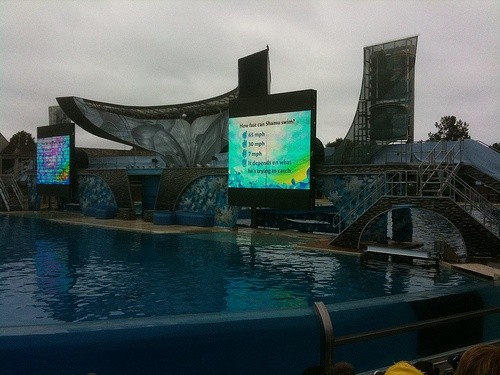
63 203 81 219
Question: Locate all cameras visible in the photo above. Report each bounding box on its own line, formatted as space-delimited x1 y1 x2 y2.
448 354 460 370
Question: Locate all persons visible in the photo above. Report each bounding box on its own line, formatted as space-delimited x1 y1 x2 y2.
454 345 500 375
303 360 439 375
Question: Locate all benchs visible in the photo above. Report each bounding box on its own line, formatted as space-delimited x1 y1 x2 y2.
86 207 215 227
